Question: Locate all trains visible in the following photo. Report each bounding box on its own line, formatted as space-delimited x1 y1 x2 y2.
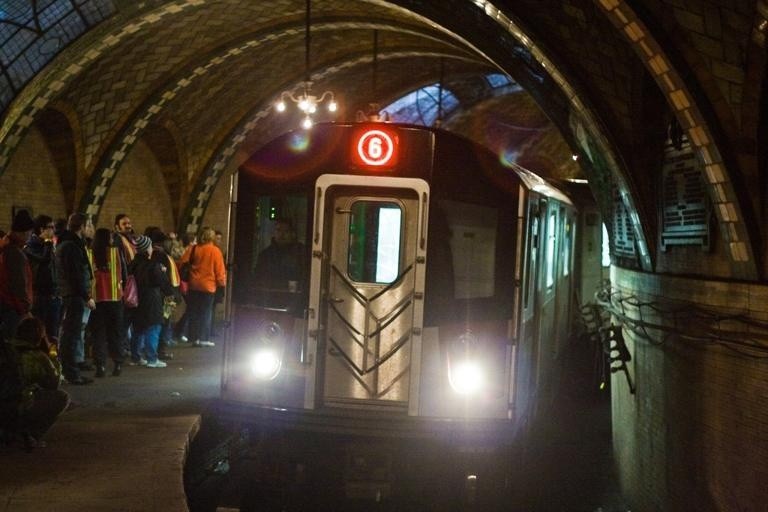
218 122 580 440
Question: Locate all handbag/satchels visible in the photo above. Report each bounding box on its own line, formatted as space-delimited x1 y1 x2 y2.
180 262 190 296
167 254 181 288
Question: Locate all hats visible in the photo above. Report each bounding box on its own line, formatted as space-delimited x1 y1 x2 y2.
10 209 35 234
17 318 47 344
134 236 152 253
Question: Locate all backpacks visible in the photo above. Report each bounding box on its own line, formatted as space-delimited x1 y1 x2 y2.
122 262 145 309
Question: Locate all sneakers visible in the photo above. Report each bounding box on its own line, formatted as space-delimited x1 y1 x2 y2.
24 419 48 449
66 335 215 384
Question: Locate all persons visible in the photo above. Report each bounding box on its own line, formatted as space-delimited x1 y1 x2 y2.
0 210 227 448
254 218 306 277
